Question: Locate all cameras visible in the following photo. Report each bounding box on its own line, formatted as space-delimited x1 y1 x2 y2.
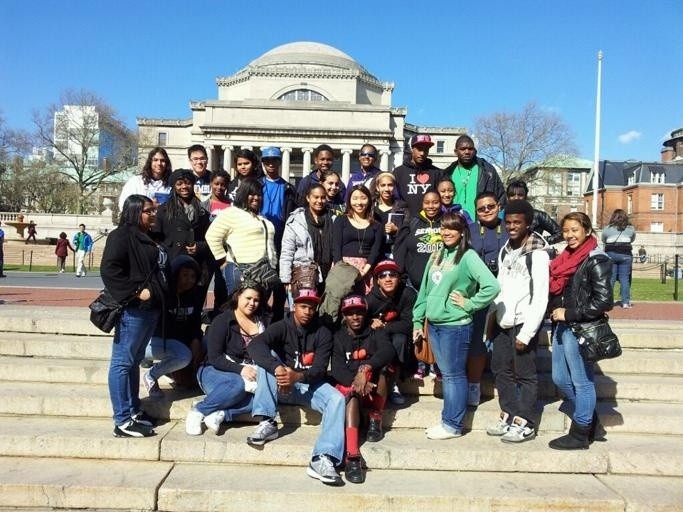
412 333 422 346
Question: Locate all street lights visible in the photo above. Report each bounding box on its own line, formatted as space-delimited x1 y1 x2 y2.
590 48 602 229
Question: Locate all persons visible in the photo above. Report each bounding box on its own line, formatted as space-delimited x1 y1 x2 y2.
330 294 397 483
24 220 36 244
118 134 564 411
99 194 169 438
54 232 74 273
0 221 6 278
545 212 613 450
412 214 500 439
487 199 550 442
245 290 345 486
601 209 635 309
71 223 93 277
184 280 267 435
637 245 646 262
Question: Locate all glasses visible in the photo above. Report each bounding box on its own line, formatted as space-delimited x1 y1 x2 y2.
143 207 157 215
264 159 280 165
343 310 364 316
359 152 375 157
192 157 207 161
477 204 497 213
378 271 400 279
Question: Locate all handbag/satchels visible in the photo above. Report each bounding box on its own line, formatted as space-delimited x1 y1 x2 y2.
172 362 202 387
291 265 318 294
239 363 257 393
414 319 436 364
347 360 378 378
570 314 622 365
241 257 280 290
88 291 123 333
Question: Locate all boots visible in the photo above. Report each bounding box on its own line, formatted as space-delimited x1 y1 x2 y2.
549 419 589 449
590 409 606 439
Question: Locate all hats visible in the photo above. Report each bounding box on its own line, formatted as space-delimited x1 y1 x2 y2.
374 260 400 274
238 279 262 294
168 169 195 186
262 147 282 158
411 134 434 147
294 288 321 305
341 295 366 311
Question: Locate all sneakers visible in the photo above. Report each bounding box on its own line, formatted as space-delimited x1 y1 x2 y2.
436 373 443 381
131 411 157 426
345 455 364 483
501 416 535 443
413 369 424 380
366 407 383 442
388 381 405 405
112 420 154 437
468 382 481 407
186 410 203 435
487 412 510 435
623 304 631 309
425 424 462 439
247 420 279 446
76 272 80 277
204 411 225 434
307 455 343 486
143 373 164 398
82 271 86 277
141 359 152 368
